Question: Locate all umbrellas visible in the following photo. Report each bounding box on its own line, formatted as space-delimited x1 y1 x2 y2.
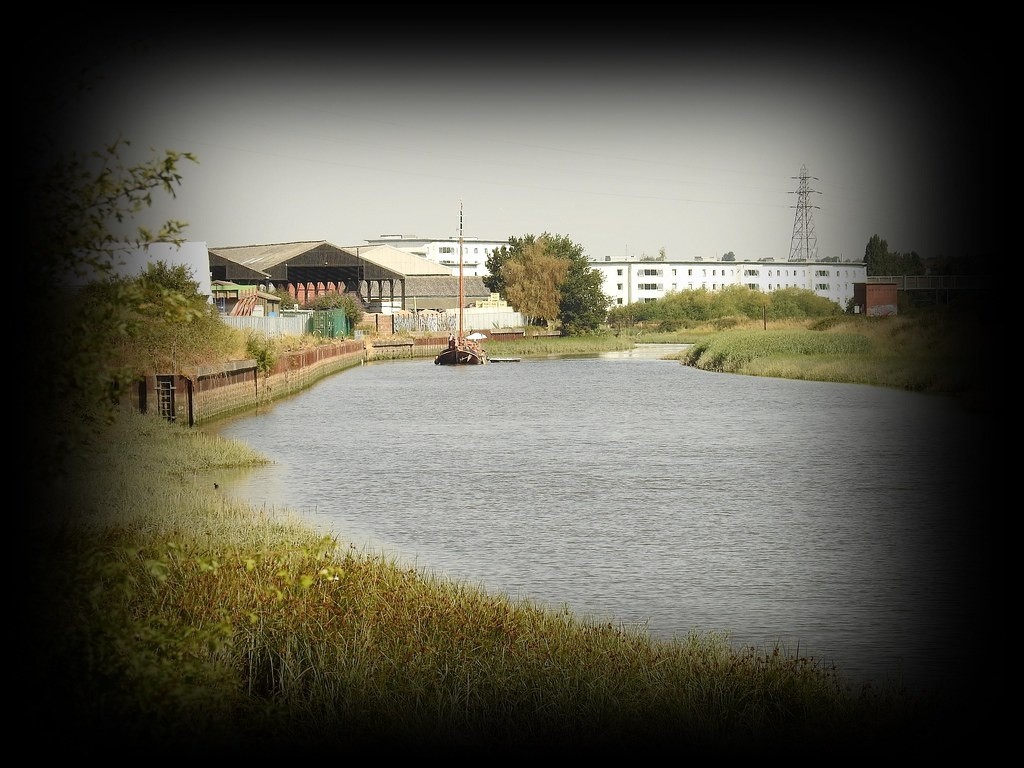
465 332 488 342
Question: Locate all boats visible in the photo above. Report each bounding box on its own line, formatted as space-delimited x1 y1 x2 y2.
435 200 482 366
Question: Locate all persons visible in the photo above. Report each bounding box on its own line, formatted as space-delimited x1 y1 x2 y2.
447 333 489 365
